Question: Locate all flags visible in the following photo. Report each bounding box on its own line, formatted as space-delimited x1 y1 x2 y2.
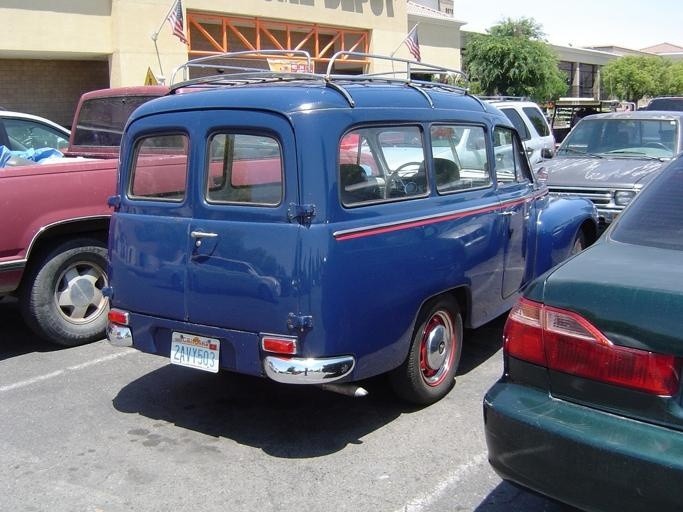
164 0 194 49
402 20 422 65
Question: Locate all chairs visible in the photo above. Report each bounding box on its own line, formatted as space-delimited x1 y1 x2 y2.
340 163 368 204
418 158 460 192
